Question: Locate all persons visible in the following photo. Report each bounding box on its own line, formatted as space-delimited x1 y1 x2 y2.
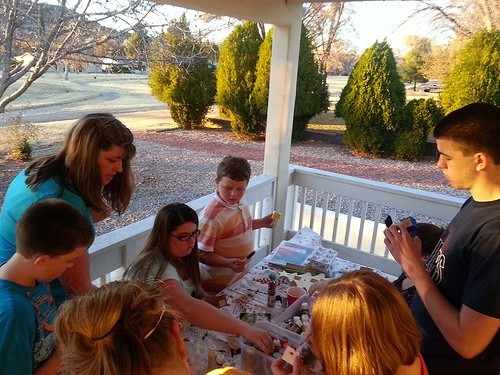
0 113 136 367
54 280 252 375
384 102 500 375
272 269 428 375
123 203 274 354
197 156 277 295
0 199 95 375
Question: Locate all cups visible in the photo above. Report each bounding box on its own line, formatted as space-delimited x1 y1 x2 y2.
286 285 306 306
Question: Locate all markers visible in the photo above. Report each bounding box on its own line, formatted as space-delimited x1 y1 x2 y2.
242 251 256 261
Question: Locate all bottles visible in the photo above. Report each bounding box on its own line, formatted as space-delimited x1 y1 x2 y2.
207 345 225 369
267 273 277 306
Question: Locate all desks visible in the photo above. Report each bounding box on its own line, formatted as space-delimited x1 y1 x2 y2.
177 239 398 375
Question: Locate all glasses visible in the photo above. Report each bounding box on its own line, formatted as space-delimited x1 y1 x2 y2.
170 230 201 241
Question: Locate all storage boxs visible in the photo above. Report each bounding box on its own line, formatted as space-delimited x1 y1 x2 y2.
237 321 304 375
275 294 315 335
